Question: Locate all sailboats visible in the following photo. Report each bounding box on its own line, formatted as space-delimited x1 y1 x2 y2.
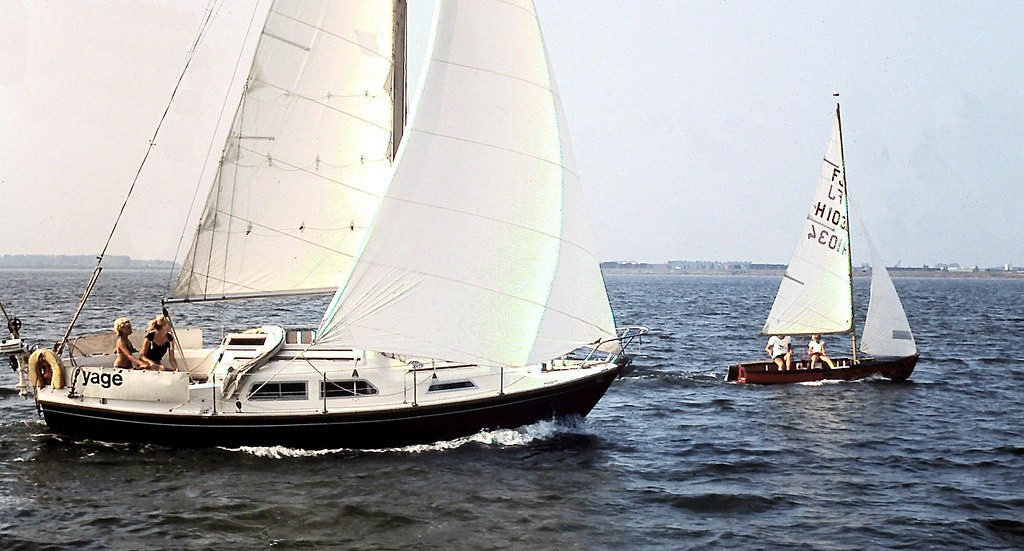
726 91 920 386
0 0 649 454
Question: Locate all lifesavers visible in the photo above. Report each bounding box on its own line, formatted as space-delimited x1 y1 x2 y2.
27 348 65 389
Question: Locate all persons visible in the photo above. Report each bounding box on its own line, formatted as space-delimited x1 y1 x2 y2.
765 334 793 371
808 333 836 370
113 317 148 369
137 315 180 372
